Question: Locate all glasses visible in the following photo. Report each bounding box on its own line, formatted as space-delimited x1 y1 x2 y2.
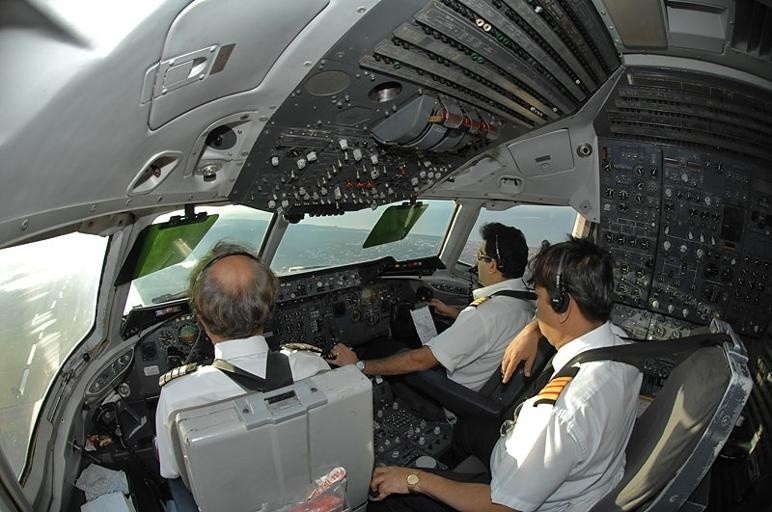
478 250 491 261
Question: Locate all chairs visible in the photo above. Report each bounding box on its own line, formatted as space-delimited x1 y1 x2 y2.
398 338 556 418
452 318 753 512
168 364 375 512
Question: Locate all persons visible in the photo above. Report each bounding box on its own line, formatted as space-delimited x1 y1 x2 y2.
155 238 331 479
370 234 643 512
327 222 536 422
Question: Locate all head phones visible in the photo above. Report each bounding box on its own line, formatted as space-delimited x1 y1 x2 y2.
550 241 570 314
493 226 511 273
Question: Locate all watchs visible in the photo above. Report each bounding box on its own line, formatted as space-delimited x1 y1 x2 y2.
406 469 422 493
356 360 365 371
